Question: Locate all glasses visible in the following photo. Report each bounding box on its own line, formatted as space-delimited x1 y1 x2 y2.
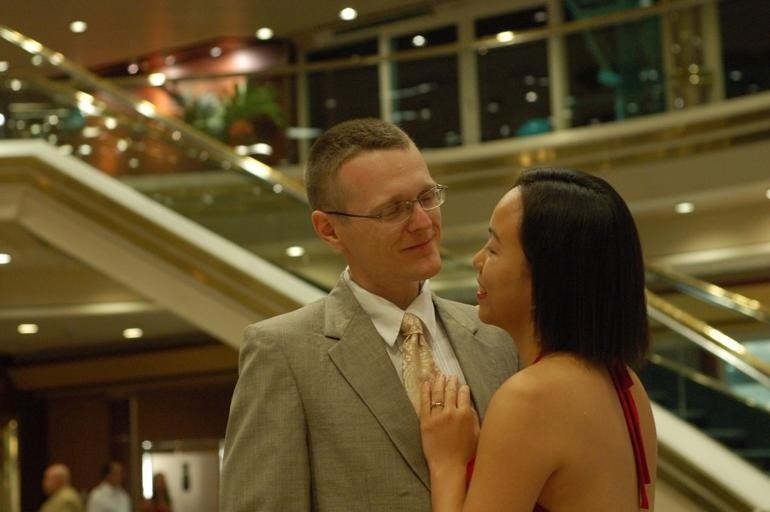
319 182 447 228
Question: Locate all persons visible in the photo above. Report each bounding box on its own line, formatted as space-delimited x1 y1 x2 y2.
38 463 85 512
421 166 658 512
221 117 518 512
152 473 173 512
85 460 133 512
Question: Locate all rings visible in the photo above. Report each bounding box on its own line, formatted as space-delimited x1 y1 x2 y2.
431 402 444 411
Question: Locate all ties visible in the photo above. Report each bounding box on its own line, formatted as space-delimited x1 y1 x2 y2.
400 312 441 419
